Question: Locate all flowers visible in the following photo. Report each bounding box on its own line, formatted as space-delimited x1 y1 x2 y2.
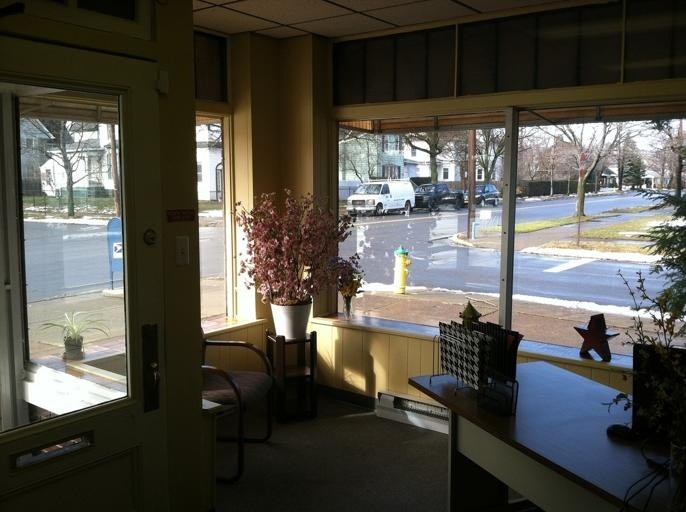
234 188 367 305
340 280 362 296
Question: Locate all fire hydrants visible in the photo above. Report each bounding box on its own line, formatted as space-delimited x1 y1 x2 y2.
393 245 411 294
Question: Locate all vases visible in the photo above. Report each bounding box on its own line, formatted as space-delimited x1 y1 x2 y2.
271 303 313 342
343 297 353 320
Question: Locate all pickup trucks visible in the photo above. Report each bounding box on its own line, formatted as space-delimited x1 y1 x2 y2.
416 183 464 210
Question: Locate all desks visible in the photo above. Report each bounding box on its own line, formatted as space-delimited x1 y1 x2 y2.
263 328 316 426
408 360 686 512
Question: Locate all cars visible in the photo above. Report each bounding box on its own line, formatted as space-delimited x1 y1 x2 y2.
464 185 500 207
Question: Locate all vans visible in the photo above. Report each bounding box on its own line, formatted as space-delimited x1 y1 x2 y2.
346 181 415 216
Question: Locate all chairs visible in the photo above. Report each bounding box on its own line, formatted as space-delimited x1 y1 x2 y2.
196 327 271 485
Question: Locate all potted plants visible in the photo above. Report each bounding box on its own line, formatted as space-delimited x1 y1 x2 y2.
601 268 686 512
41 309 109 358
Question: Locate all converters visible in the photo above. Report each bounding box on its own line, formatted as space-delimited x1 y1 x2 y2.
645 456 679 480
